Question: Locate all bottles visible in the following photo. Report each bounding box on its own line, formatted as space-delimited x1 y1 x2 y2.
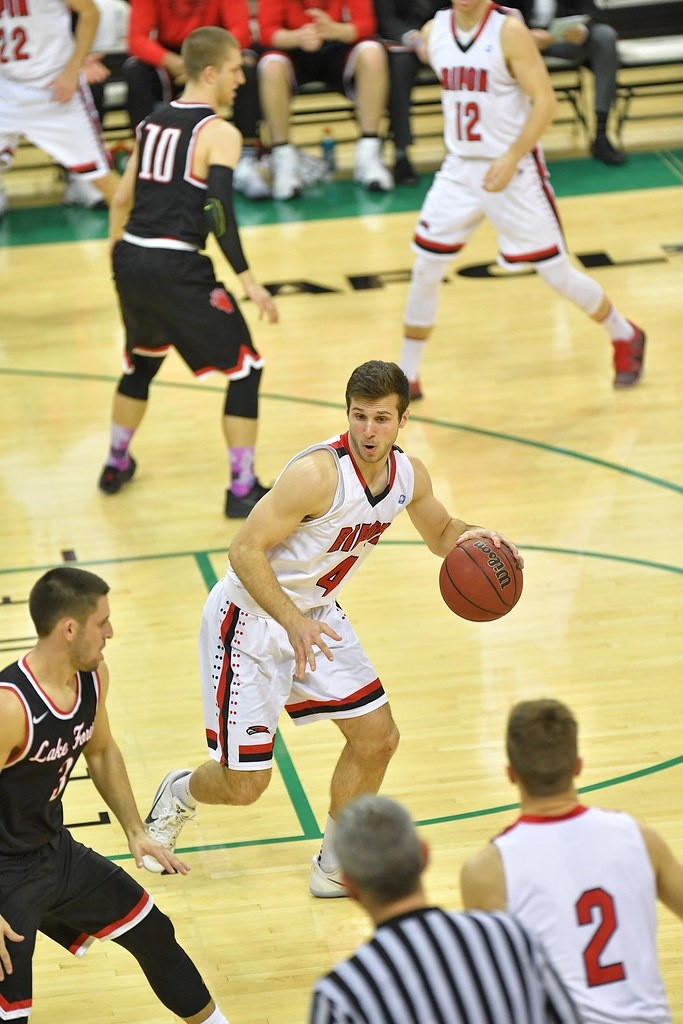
318 127 336 172
113 139 131 176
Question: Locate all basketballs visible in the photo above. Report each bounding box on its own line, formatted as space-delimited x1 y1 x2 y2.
438 536 524 623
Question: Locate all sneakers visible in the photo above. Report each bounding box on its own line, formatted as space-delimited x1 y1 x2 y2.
409 381 423 400
226 480 272 518
99 456 136 495
140 769 196 874
309 854 350 898
612 319 646 385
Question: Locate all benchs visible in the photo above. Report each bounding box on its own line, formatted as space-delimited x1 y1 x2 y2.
0 35 683 183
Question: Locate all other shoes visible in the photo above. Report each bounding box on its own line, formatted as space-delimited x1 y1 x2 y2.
61 176 103 208
590 135 625 165
393 155 419 186
352 137 394 191
271 146 302 199
233 159 269 198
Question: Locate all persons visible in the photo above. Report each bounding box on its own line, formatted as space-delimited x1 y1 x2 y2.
142 360 524 898
99 26 279 520
0 0 626 214
461 698 683 1024
0 567 232 1024
309 796 585 1024
396 0 646 401
